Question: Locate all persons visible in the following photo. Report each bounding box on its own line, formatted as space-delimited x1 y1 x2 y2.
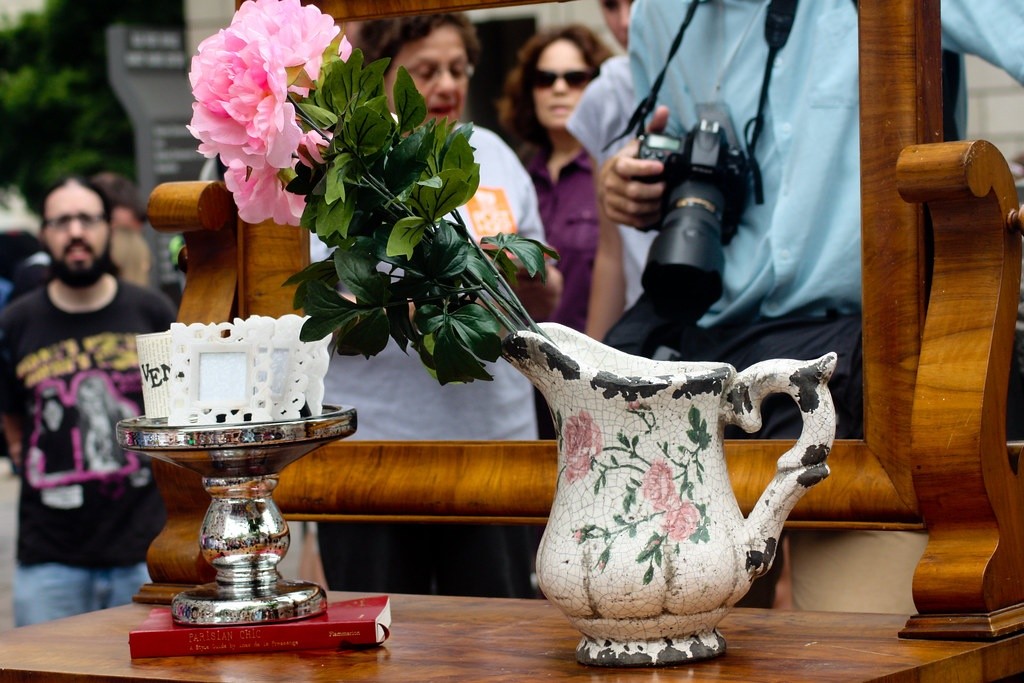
0 0 1024 608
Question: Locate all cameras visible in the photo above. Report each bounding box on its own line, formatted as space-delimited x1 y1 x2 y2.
640 119 755 307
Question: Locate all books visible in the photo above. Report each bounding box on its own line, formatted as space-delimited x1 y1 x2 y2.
128 595 393 660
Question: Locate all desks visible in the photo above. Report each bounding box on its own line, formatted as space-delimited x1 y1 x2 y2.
0 591 1024 683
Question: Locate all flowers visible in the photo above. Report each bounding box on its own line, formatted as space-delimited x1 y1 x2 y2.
185 0 558 389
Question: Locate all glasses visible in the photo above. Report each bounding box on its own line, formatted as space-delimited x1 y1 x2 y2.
386 59 476 81
45 212 106 231
532 68 595 87
